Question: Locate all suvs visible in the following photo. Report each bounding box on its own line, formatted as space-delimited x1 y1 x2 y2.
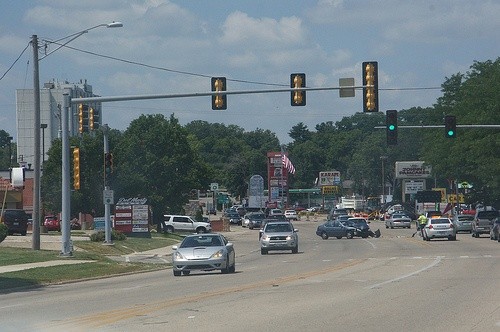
162 214 212 234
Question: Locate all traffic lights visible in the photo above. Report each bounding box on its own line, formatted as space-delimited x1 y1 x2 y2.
104 153 111 187
78 105 89 133
90 109 100 130
73 147 86 190
445 117 456 137
387 110 397 144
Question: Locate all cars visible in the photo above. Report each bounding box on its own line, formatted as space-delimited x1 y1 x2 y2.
315 220 366 239
259 222 299 254
299 206 370 230
44 216 61 233
417 209 456 242
385 214 412 229
226 205 298 229
473 210 500 243
205 208 217 215
172 233 235 276
452 214 473 234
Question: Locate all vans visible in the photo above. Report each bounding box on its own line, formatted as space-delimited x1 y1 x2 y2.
0 209 28 236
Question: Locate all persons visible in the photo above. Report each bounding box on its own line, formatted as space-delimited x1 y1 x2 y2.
417 213 428 236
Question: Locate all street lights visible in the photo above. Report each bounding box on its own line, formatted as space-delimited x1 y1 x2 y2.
41 123 47 161
30 22 123 251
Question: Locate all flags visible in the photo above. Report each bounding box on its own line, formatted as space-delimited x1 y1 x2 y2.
282 148 295 175
